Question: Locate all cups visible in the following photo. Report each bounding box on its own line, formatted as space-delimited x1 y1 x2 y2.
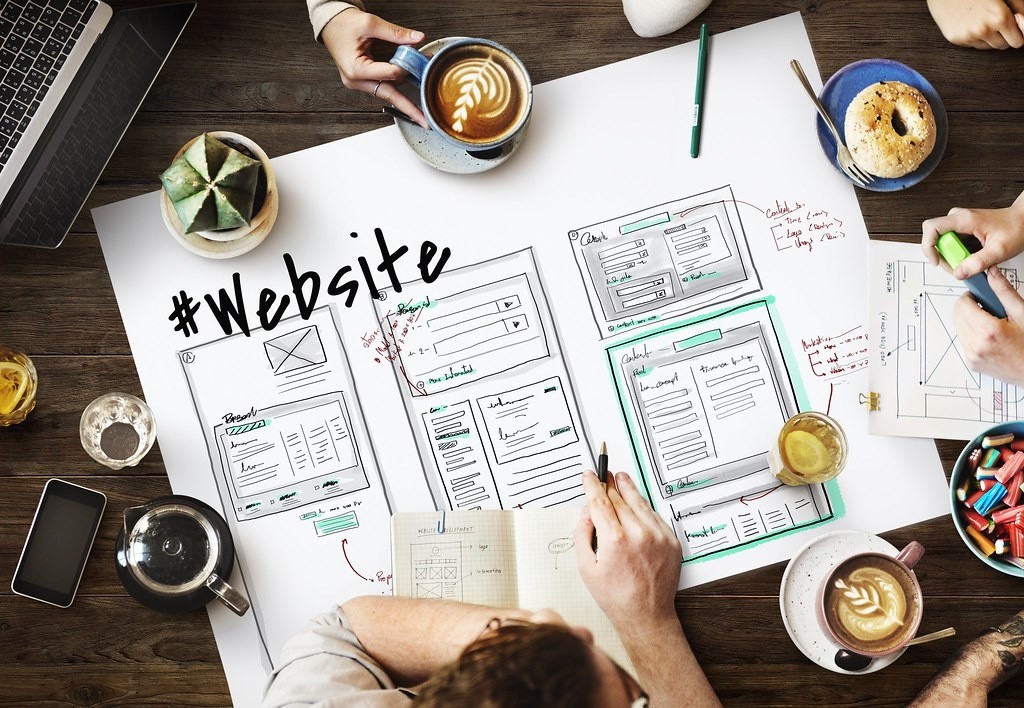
79 392 157 469
820 541 926 656
770 411 847 485
390 39 535 149
0 344 37 427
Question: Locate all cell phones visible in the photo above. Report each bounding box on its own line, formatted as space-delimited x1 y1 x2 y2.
11 478 108 607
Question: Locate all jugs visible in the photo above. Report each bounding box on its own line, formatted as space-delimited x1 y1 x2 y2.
114 495 251 617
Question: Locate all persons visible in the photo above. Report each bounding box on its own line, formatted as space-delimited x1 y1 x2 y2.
907 609 1024 708
306 0 713 130
921 189 1024 389
927 0 1024 50
261 470 722 708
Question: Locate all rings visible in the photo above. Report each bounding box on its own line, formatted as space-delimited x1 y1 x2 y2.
371 80 383 97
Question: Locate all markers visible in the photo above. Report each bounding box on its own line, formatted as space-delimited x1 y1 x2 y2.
938 231 1007 319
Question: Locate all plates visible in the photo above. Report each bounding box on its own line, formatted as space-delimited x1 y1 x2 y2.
158 178 279 260
780 530 924 676
394 34 529 174
817 59 948 191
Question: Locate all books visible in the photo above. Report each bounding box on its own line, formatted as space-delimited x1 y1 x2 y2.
390 511 639 681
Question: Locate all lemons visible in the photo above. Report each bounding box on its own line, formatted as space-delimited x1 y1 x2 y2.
0 361 28 414
785 430 828 474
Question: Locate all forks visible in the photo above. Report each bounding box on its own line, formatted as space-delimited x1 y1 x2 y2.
791 59 876 187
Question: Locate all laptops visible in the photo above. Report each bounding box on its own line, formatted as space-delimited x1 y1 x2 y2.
0 0 198 249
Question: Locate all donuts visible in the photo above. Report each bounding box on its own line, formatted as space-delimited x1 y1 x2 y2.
844 80 936 178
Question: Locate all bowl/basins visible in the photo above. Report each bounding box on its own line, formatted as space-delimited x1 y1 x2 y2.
950 420 1024 577
169 130 275 240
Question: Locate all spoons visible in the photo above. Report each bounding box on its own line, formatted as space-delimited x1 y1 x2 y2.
383 106 503 159
836 628 956 670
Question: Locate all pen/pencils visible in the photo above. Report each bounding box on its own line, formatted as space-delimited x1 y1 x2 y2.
690 22 709 158
592 441 609 563
